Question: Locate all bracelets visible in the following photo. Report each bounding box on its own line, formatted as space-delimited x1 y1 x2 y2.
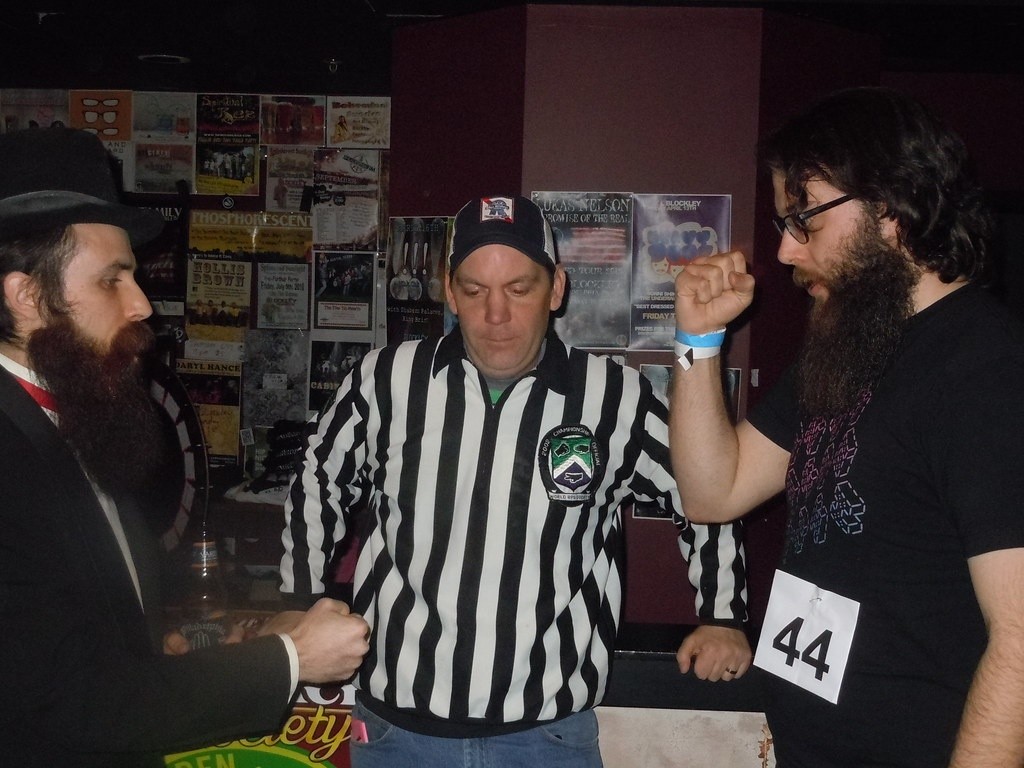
674 327 726 370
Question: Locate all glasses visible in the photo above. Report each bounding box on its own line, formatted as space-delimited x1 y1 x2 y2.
774 189 866 244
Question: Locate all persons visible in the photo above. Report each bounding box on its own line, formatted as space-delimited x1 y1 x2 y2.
249 189 753 768
671 80 1024 767
0 126 370 768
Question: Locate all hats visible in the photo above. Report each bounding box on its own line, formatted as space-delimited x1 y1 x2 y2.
447 191 555 283
0 128 164 249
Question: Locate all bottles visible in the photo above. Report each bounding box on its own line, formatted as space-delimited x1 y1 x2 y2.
180 527 234 650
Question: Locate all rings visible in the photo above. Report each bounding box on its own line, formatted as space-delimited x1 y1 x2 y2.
725 668 737 674
